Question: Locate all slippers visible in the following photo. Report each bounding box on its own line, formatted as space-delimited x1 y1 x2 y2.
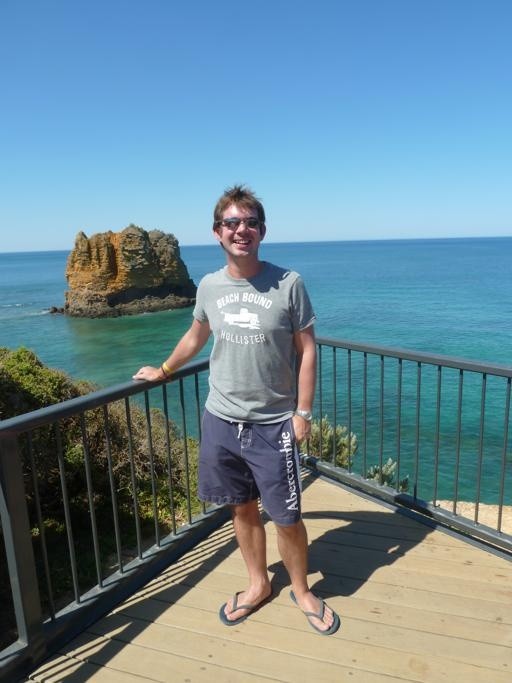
289 589 340 636
219 590 274 626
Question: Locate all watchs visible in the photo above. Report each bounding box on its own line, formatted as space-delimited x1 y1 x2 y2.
293 409 313 421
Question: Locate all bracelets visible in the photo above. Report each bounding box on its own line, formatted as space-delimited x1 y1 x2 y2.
161 365 168 377
163 361 172 374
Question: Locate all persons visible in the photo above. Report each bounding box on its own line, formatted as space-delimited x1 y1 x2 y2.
132 183 341 635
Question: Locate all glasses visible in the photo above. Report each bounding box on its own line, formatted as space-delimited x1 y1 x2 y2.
221 217 258 228
